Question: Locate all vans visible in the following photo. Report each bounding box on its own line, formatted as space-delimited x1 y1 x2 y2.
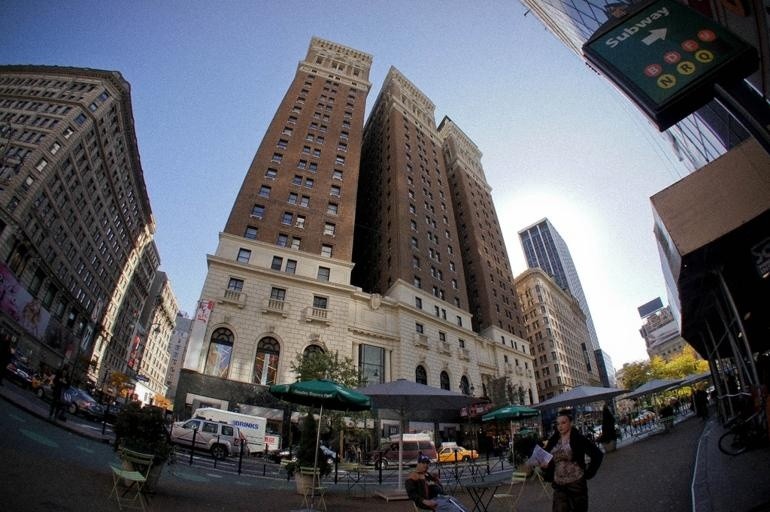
191 407 266 453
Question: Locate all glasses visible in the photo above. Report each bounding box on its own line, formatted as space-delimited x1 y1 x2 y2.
556 411 573 417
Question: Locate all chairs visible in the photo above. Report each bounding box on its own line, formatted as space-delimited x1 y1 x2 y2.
298 466 329 512
272 458 292 481
107 447 155 511
493 471 527 512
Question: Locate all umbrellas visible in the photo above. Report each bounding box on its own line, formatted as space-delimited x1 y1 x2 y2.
265 378 371 512
616 379 687 415
481 404 539 466
530 384 632 436
353 378 489 491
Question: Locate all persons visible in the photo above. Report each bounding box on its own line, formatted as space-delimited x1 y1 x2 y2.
405 455 470 512
49 378 73 419
658 350 770 431
539 410 603 512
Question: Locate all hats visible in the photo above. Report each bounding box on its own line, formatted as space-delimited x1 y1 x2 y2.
418 455 431 463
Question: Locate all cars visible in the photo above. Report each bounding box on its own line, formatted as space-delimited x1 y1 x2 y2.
7 361 122 424
365 434 479 469
631 409 655 427
171 419 247 458
268 443 340 470
594 423 622 445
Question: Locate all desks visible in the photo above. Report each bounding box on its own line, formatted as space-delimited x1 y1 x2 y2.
441 463 503 512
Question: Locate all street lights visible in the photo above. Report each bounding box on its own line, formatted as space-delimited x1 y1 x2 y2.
135 319 162 373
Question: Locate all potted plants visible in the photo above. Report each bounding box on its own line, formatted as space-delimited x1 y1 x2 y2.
657 404 674 429
506 431 547 476
108 399 178 493
284 437 333 496
595 426 622 453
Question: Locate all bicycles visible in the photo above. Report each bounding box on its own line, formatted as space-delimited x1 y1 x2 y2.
716 402 770 455
